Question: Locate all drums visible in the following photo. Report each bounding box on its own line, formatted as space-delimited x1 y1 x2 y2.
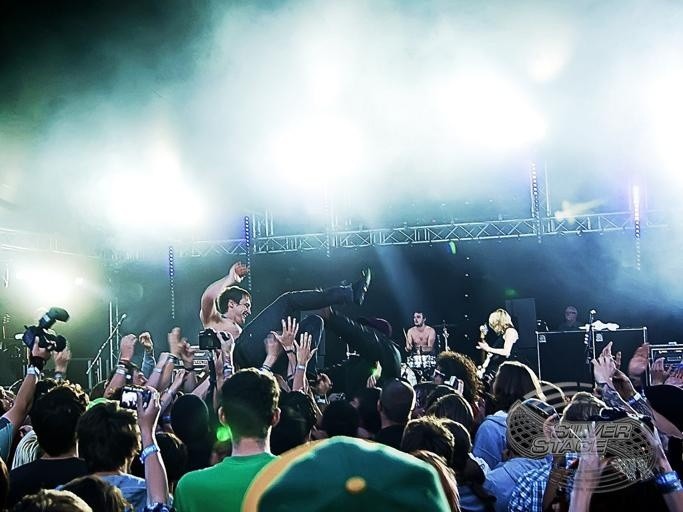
407 355 437 368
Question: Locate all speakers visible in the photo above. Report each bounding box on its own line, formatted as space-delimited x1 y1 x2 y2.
505 297 540 382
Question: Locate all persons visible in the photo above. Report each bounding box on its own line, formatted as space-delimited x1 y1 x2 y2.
0 262 681 512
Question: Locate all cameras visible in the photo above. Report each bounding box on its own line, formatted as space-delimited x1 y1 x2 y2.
589 407 656 459
120 385 151 410
22 307 69 352
199 327 230 350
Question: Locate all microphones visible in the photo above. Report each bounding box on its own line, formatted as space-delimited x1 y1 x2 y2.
116 313 128 327
588 308 596 322
442 327 449 338
